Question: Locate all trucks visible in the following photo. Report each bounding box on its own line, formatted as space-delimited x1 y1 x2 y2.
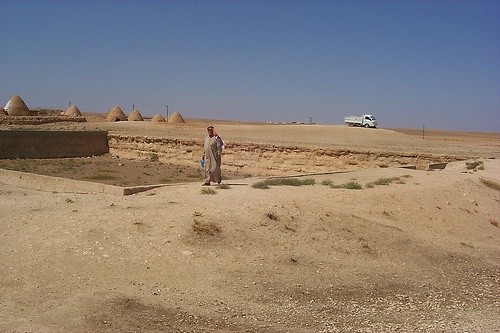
344 114 377 128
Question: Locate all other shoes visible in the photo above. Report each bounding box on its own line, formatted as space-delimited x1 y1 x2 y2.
218 179 221 183
202 182 210 186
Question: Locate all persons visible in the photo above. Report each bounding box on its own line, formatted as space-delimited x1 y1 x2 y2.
202 126 224 186
